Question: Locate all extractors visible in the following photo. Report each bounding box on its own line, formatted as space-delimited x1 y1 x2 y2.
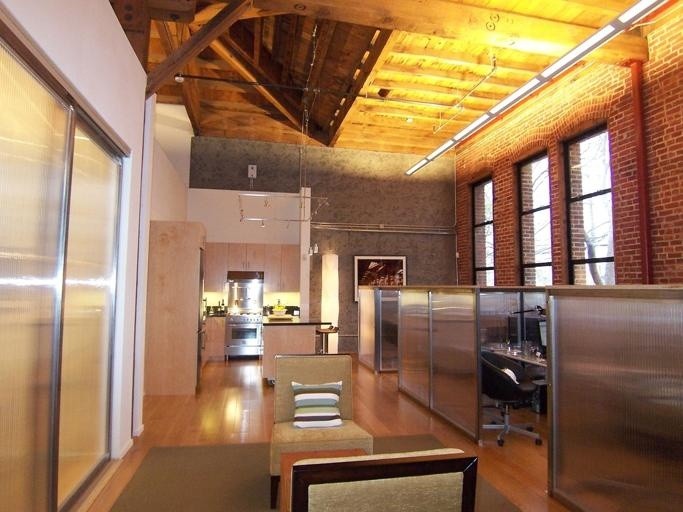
227 271 264 283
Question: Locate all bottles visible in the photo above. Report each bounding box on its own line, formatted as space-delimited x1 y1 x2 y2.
217 301 221 317
221 299 225 317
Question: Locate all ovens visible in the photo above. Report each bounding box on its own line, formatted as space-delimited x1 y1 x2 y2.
224 323 264 361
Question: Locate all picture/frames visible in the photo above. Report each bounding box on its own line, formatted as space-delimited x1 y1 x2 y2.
354 254 407 304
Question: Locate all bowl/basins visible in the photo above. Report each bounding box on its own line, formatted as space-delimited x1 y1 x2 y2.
272 309 288 316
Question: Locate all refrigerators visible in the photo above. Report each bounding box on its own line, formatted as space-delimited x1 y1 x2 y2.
196 247 207 394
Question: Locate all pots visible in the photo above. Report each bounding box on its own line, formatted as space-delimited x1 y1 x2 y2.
235 298 258 313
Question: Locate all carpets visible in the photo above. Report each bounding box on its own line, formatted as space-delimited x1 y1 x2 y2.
111 434 524 511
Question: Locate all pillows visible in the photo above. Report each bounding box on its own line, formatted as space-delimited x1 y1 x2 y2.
288 377 343 427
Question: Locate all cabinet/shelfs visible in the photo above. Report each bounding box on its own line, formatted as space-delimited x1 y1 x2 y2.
207 316 226 362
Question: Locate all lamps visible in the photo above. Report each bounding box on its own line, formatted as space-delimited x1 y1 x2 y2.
320 248 340 354
306 243 318 259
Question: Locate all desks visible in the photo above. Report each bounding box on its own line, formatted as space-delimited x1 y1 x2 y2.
489 342 546 373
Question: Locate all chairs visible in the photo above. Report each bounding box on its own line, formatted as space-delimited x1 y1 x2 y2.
289 449 477 511
268 353 374 510
480 349 543 446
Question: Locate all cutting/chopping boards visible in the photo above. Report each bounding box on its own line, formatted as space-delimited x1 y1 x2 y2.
268 315 293 320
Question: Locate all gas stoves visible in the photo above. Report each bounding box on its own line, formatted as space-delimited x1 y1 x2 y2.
225 313 263 324
